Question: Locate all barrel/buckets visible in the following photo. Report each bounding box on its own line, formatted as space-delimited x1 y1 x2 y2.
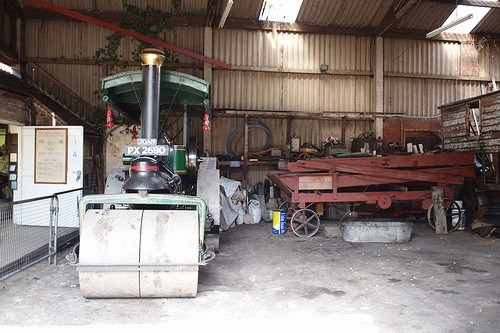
272 209 287 234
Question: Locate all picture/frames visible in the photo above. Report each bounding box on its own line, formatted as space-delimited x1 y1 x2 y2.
34 128 68 184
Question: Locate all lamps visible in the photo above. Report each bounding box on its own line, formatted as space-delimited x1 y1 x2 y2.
320 65 328 72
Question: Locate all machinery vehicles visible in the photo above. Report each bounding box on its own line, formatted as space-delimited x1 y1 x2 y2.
74 48 217 295
